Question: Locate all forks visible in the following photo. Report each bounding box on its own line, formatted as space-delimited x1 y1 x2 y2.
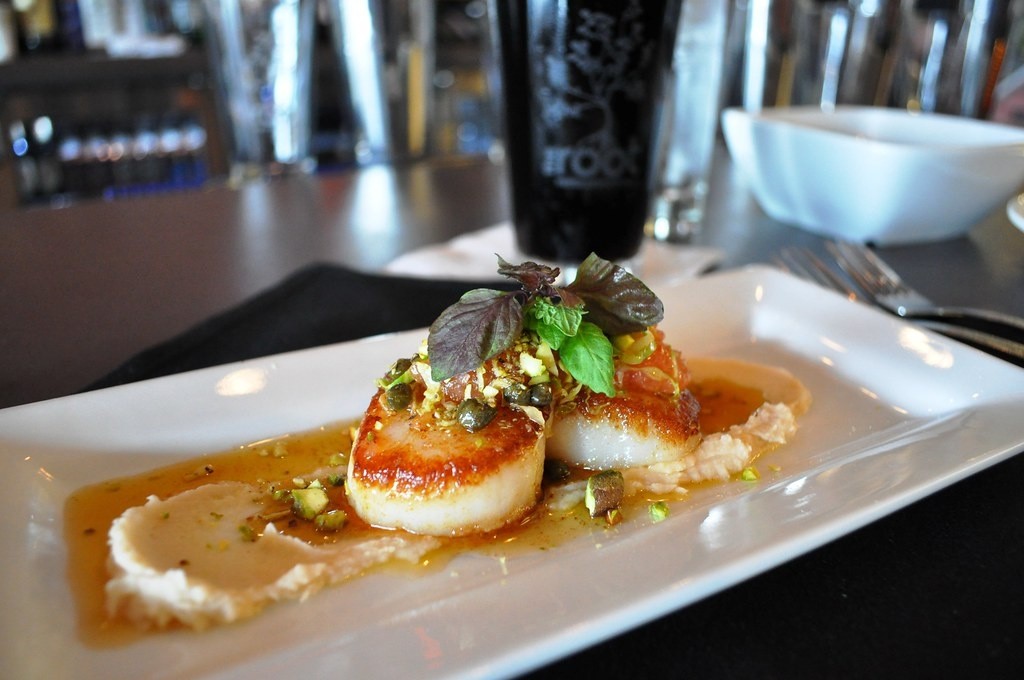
821 235 1024 336
766 240 1024 364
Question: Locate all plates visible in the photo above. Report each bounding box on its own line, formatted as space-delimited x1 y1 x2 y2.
0 265 1024 680
1005 192 1024 233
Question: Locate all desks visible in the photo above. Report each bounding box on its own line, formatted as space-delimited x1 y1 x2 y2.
0 157 1024 680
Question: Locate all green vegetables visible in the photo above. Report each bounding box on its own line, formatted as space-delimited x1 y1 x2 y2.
428 251 665 397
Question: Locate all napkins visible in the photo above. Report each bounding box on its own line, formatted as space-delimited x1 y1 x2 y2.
386 217 724 281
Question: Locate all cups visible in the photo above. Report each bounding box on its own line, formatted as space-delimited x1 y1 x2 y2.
638 2 749 244
198 0 318 169
790 2 899 104
890 0 1014 118
333 0 438 164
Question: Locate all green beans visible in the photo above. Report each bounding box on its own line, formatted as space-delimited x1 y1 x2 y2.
386 382 552 431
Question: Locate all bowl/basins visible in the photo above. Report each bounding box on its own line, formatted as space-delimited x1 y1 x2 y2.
718 95 1024 248
